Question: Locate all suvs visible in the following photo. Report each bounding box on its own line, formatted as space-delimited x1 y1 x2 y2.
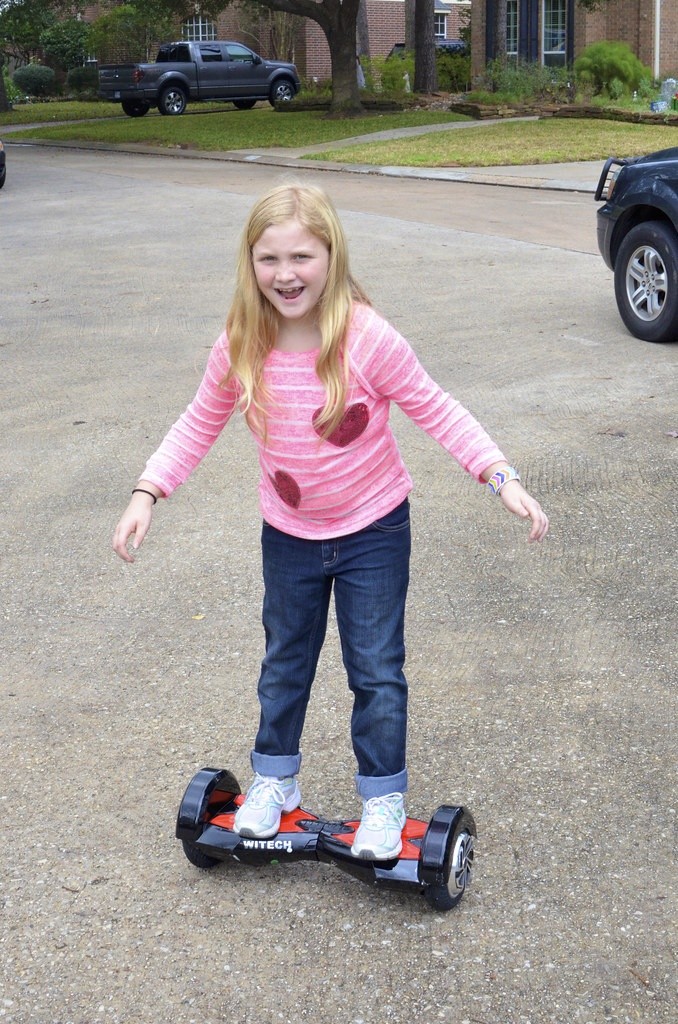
381 41 469 93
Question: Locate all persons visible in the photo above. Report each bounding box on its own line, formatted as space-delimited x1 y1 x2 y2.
112 185 549 861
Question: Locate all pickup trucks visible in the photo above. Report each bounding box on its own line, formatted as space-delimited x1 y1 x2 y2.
97 39 302 117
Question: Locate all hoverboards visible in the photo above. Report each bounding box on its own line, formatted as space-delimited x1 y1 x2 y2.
174 767 478 910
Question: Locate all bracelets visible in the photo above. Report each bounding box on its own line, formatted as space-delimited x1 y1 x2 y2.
487 467 521 495
131 489 156 505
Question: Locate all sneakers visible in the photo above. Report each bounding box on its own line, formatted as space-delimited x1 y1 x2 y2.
232 772 301 840
350 792 407 863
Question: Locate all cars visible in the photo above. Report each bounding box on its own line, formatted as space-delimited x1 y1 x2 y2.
595 148 678 343
